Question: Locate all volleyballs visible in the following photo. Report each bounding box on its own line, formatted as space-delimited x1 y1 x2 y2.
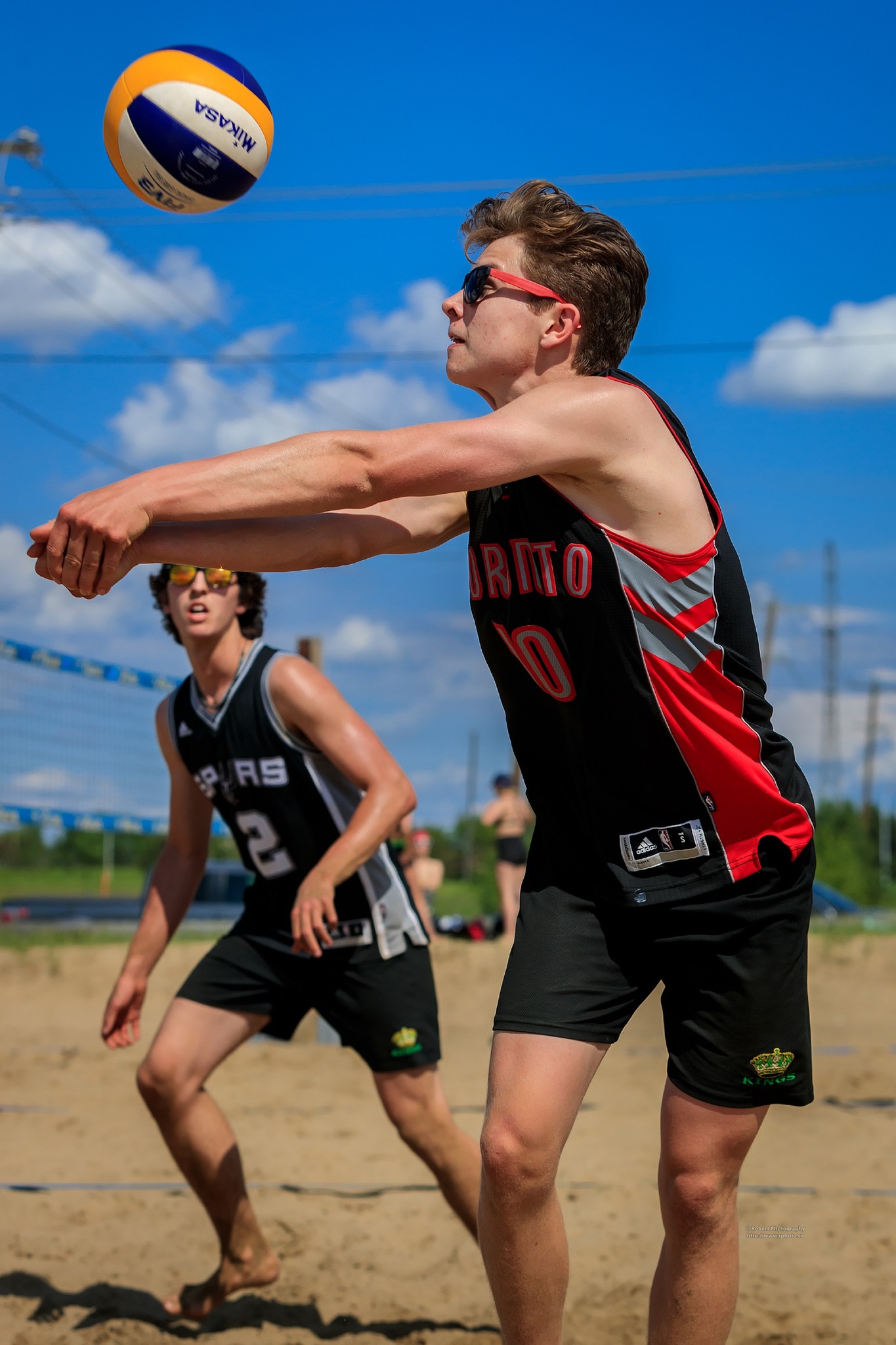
102 44 275 213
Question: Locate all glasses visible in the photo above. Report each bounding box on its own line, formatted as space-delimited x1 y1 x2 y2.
169 565 241 589
461 265 584 330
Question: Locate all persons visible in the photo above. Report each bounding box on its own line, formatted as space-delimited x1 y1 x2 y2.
483 774 535 948
25 180 816 1345
100 564 484 1317
388 814 445 952
